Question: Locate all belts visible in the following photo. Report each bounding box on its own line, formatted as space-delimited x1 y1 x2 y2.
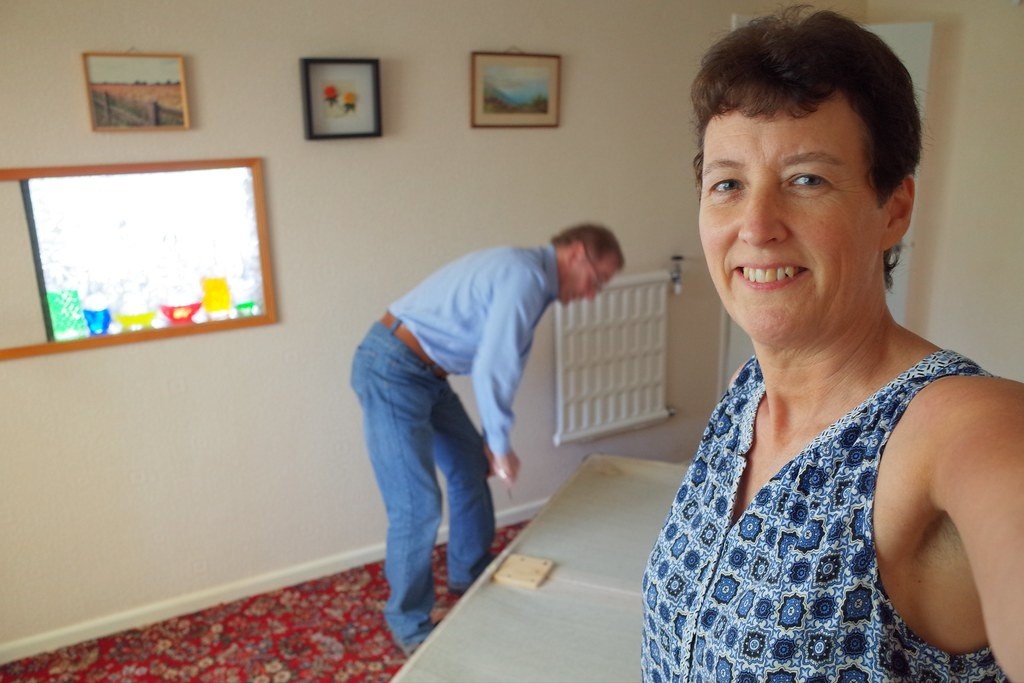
381 310 451 382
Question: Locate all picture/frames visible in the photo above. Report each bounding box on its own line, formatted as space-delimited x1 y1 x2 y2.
80 52 191 133
470 51 562 128
299 57 382 141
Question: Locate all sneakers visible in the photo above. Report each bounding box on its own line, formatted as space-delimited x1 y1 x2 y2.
390 627 425 658
447 577 471 595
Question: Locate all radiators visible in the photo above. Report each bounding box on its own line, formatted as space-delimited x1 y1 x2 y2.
553 254 684 447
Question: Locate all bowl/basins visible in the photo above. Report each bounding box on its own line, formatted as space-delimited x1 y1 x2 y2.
113 310 155 332
160 301 203 324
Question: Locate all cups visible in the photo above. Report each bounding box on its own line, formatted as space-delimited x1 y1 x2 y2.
83 308 112 336
203 277 234 321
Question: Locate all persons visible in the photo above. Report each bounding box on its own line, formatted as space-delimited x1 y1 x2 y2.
353 223 626 657
641 9 1024 683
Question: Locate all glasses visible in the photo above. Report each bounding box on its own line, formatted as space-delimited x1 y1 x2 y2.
583 243 604 292
